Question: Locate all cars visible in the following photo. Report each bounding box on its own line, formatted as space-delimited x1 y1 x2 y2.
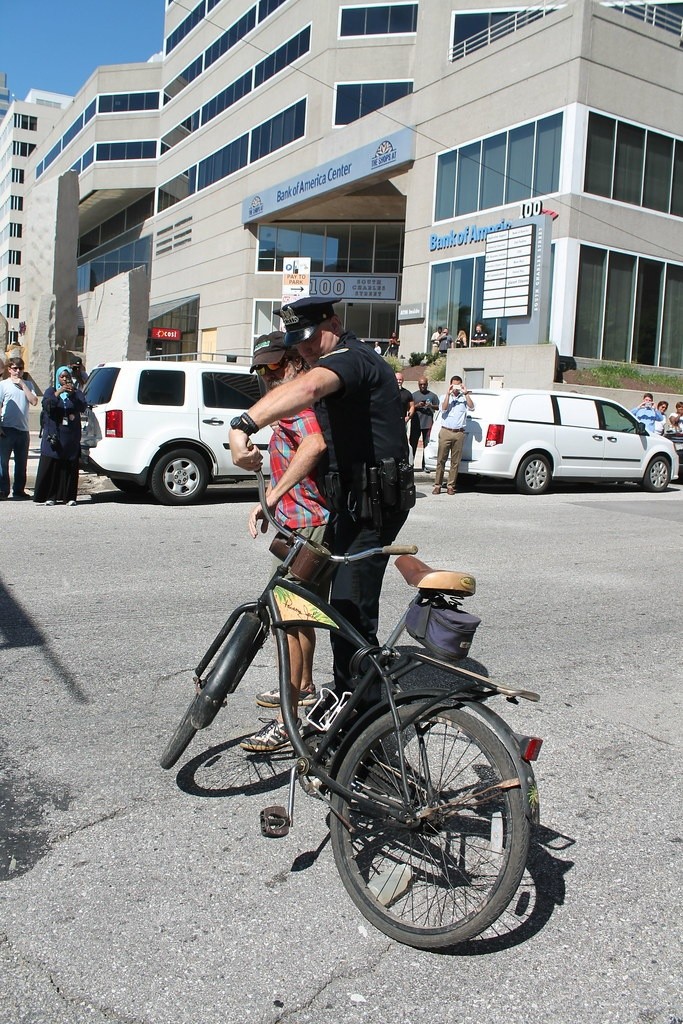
663 433 683 482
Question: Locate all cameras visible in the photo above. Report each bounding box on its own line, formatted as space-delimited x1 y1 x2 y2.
47 434 58 447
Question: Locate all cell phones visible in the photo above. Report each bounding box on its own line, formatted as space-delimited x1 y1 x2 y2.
645 403 651 407
453 385 462 389
421 400 426 406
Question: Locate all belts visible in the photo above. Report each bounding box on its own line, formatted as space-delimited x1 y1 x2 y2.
442 426 465 432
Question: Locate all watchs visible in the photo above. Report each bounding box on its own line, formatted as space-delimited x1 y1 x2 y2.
230 416 253 436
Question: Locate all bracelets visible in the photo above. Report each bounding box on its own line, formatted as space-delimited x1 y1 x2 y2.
465 392 469 395
241 412 259 434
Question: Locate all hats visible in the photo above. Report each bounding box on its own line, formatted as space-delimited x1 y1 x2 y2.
71 356 82 364
272 297 342 345
249 331 290 374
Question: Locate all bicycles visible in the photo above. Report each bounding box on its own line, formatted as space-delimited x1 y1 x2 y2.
161 464 542 952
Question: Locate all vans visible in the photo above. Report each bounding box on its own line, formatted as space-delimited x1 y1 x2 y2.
76 353 273 506
424 388 679 495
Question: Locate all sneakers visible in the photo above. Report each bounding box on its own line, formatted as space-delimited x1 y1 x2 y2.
255 684 316 708
240 717 303 751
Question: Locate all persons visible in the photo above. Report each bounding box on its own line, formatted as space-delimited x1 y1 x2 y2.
471 325 488 346
389 332 400 357
228 297 415 719
396 373 440 473
631 393 668 436
669 402 683 432
374 342 381 355
241 332 331 752
0 358 38 498
431 327 467 355
34 356 87 505
433 376 475 495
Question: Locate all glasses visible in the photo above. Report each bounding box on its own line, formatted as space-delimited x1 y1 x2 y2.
256 357 298 376
10 367 23 371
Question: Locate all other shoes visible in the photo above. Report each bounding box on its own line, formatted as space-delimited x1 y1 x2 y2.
13 493 34 500
45 500 56 506
446 488 454 495
432 488 441 494
303 687 352 719
422 467 431 473
66 501 76 506
